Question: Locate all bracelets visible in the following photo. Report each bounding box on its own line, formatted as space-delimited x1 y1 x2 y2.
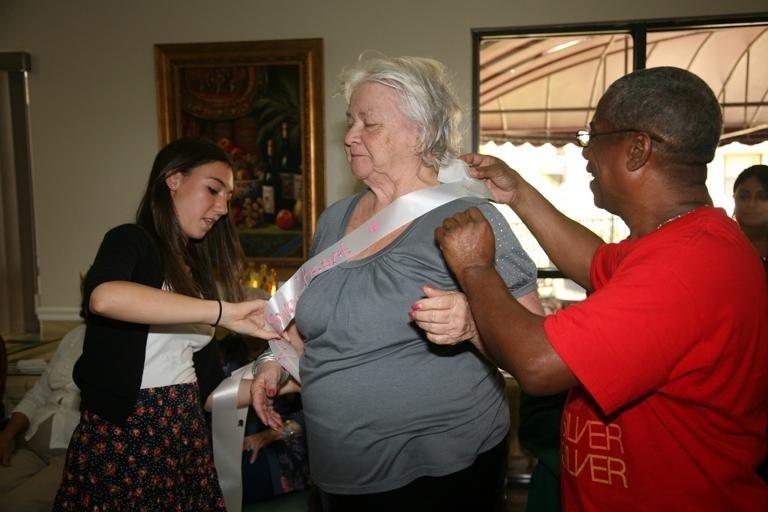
211 298 222 327
251 347 289 385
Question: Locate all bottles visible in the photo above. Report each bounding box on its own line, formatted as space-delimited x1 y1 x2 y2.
261 120 303 226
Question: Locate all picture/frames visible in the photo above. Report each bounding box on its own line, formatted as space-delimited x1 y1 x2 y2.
153 36 326 271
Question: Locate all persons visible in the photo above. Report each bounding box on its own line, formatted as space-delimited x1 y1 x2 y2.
52 134 301 512
248 47 549 511
731 164 768 264
1 264 93 511
433 64 768 511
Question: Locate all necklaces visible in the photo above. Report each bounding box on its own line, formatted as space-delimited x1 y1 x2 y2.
655 203 709 231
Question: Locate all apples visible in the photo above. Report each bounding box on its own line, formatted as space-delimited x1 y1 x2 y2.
230 146 242 157
231 207 244 224
236 168 253 180
218 137 232 152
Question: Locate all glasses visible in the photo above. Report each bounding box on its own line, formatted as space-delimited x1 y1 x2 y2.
574 129 665 148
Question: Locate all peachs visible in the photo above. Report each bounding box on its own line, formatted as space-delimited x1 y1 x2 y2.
277 209 294 230
